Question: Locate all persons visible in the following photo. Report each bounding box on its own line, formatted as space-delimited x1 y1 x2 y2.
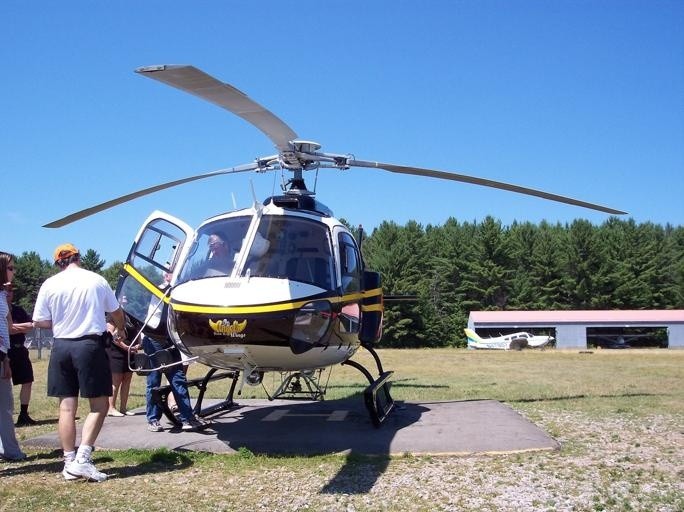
103 288 139 418
206 231 229 262
1 281 35 428
29 243 127 484
138 263 213 432
166 351 190 416
0 251 36 463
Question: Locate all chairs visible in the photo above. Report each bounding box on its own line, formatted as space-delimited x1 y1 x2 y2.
286 257 328 288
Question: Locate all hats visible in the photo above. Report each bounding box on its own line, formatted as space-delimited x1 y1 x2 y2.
54 244 77 259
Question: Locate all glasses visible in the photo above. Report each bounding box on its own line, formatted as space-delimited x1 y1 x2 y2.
7 265 14 271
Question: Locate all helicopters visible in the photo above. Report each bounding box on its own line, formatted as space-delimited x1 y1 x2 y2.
43 63 628 427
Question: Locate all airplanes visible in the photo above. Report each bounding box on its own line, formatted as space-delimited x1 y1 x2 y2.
464 328 554 349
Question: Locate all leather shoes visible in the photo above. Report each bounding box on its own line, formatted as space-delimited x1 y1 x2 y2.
109 411 135 416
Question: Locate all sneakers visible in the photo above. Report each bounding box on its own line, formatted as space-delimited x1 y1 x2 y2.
17 415 37 424
2 451 25 460
147 421 163 432
182 418 206 429
62 457 107 481
